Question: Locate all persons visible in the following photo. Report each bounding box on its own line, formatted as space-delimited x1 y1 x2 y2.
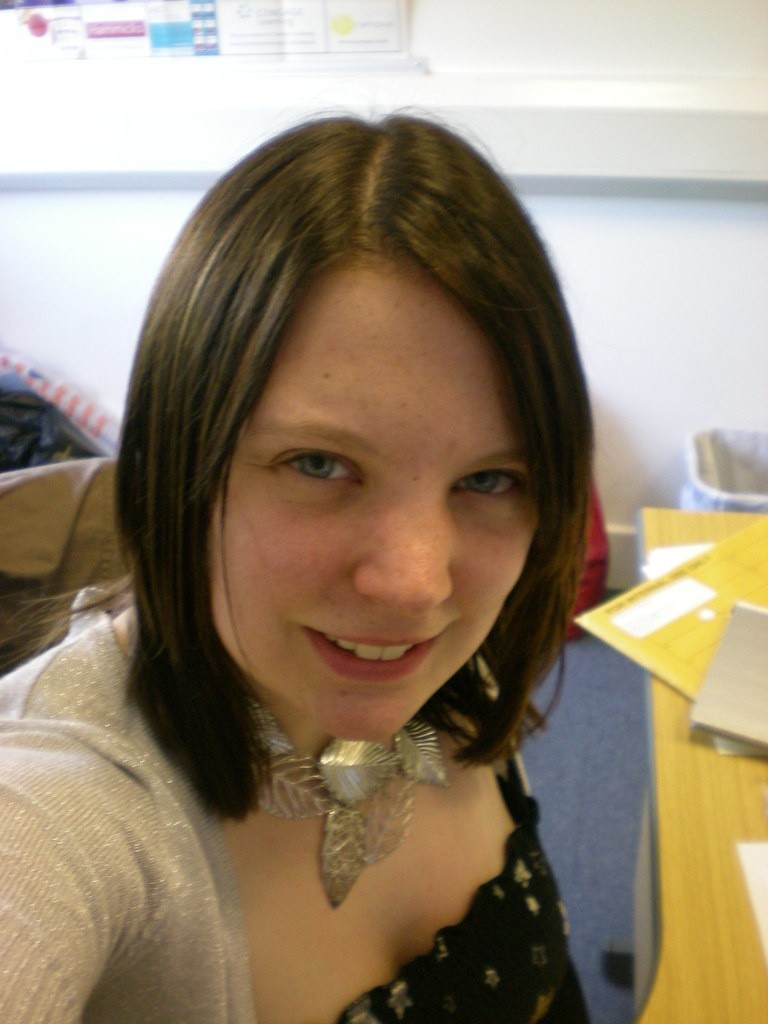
0 111 596 1024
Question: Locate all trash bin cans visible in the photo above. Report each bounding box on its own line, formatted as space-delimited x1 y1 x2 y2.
676 420 768 516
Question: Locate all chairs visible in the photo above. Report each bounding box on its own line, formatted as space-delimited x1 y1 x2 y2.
0 458 132 681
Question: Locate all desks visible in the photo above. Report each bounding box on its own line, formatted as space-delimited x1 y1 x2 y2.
637 504 768 1024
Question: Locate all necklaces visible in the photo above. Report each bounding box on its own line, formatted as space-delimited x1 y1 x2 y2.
248 691 453 910
